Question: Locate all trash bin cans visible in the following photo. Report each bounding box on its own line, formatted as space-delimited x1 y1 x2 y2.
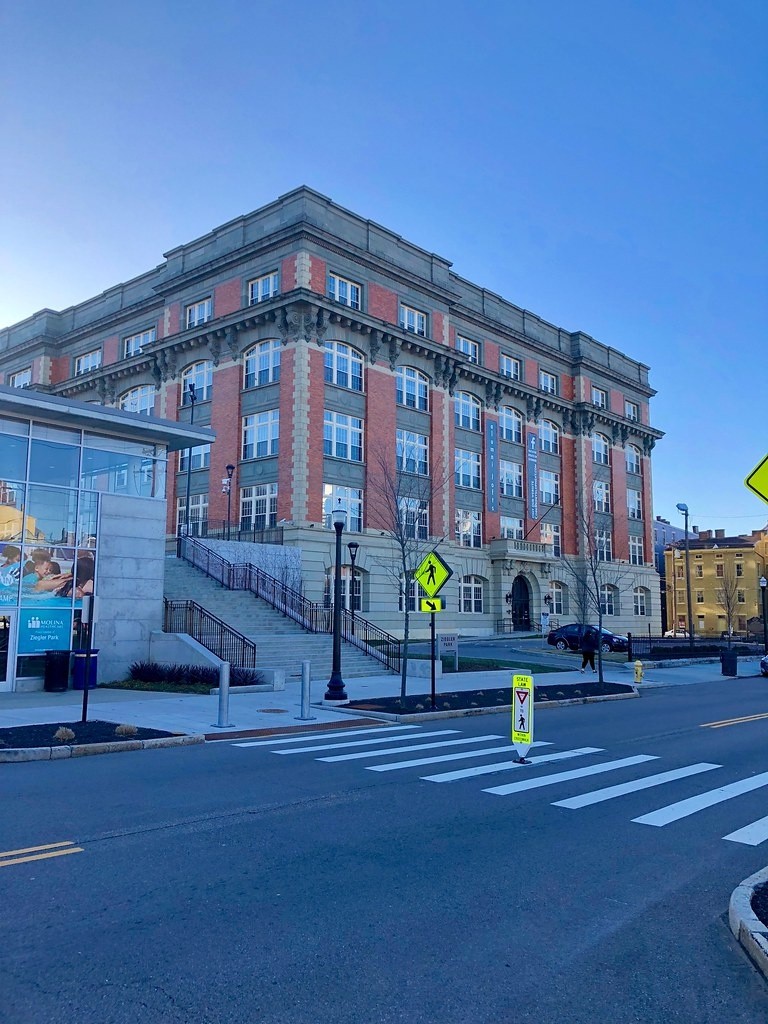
42 649 71 692
74 648 100 690
719 649 738 676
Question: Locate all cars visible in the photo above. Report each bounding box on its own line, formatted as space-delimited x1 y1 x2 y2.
664 629 699 639
547 623 635 652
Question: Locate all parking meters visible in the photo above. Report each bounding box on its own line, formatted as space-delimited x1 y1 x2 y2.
540 612 550 648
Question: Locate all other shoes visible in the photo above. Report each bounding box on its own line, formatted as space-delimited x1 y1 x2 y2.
581 669 585 674
593 670 598 674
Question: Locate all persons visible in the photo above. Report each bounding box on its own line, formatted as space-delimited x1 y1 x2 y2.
0 545 95 600
580 630 598 674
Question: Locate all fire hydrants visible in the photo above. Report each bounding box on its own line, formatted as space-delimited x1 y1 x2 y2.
633 660 645 684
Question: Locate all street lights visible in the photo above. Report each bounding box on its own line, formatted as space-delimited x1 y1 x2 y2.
347 541 359 636
672 548 680 637
759 577 768 656
325 497 349 700
225 463 235 541
185 382 198 538
677 502 695 647
